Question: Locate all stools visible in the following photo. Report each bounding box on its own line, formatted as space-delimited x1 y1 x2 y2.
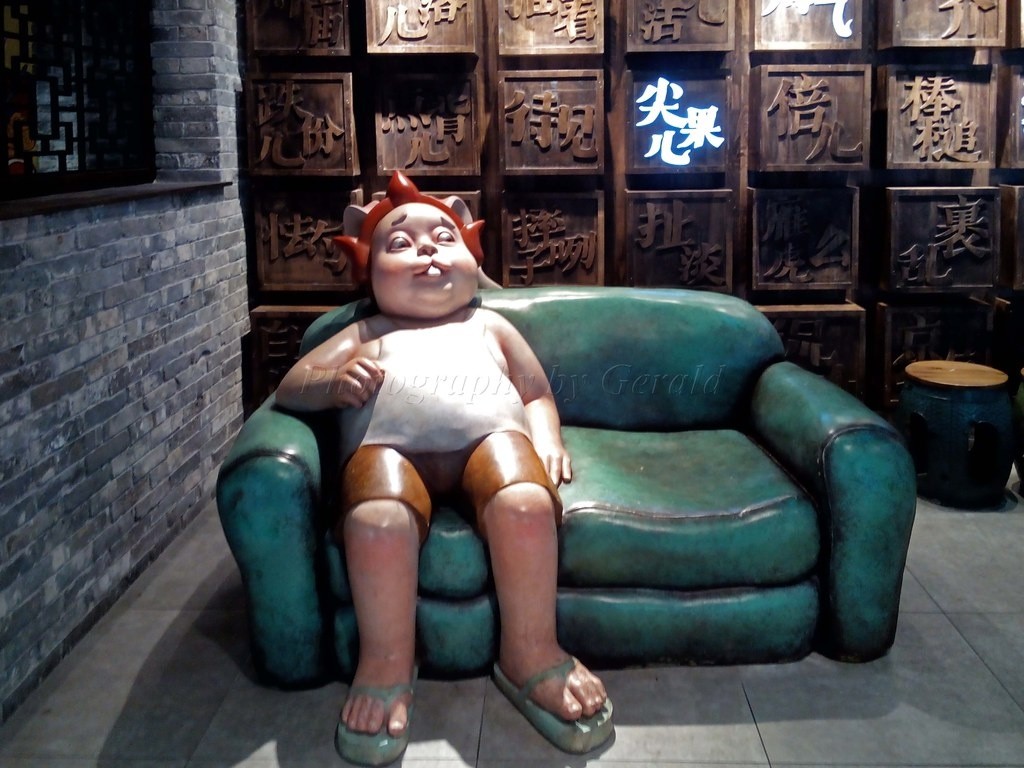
1013 367 1024 498
905 360 1014 511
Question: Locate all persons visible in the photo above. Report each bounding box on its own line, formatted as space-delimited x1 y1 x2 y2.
274 167 614 766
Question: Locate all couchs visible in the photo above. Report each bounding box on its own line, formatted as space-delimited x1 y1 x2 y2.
215 283 918 691
